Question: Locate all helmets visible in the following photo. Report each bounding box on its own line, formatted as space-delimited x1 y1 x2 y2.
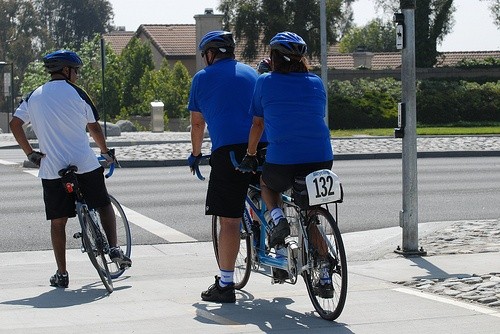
42 49 83 72
269 32 308 61
198 30 236 50
256 59 273 73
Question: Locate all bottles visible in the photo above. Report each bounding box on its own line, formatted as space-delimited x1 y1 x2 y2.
88 208 98 227
263 210 274 231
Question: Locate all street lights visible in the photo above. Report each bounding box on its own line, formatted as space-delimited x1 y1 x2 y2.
0 61 14 116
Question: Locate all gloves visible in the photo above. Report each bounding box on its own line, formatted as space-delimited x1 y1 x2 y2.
27 151 47 168
100 149 120 168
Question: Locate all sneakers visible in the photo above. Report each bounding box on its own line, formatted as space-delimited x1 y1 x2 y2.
272 266 289 283
201 275 237 303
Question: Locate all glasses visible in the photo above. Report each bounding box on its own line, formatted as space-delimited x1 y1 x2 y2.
201 48 211 57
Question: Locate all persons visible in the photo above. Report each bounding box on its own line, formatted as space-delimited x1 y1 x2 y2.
256 58 272 74
187 30 269 303
235 31 335 298
10 50 132 289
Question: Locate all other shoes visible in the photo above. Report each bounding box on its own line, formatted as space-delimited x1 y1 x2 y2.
244 244 270 263
108 245 132 264
268 217 290 248
50 269 69 288
312 281 334 299
314 252 337 272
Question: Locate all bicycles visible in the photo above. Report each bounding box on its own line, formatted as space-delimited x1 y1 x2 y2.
193 142 348 322
58 156 132 293
